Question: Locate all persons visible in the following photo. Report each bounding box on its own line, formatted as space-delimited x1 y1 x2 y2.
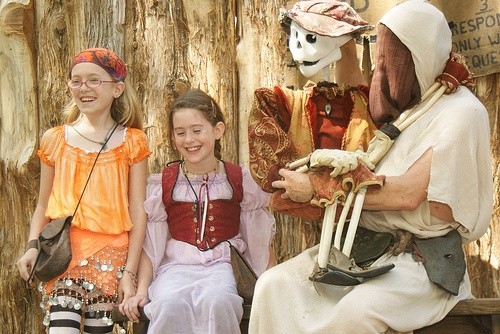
120 90 276 334
20 47 149 334
253 0 494 334
247 0 473 288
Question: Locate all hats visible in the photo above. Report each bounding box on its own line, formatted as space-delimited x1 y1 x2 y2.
69 47 127 83
279 0 376 37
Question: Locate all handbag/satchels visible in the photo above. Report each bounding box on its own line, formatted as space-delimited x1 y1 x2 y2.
35 216 73 281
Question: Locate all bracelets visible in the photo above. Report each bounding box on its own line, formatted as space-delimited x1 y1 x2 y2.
25 239 39 252
123 270 138 290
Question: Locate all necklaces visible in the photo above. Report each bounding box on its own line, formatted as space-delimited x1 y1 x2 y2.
183 162 222 209
314 85 341 117
73 123 115 149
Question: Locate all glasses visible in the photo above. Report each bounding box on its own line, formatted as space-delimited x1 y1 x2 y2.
67 77 116 89
